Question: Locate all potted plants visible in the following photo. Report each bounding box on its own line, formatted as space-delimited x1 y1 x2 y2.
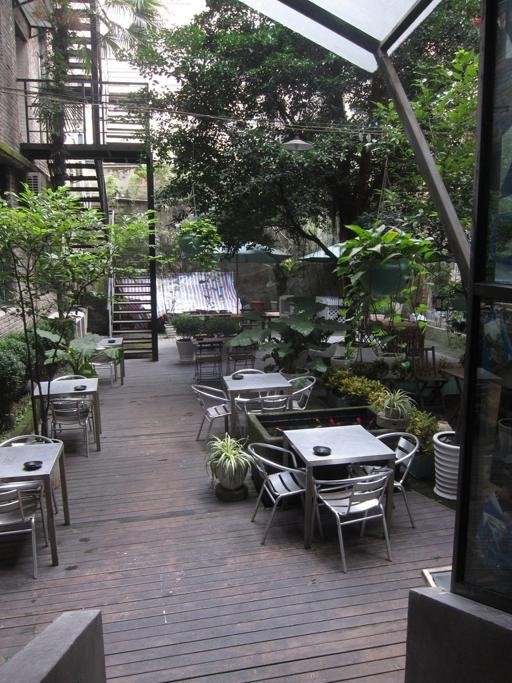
176 312 246 360
260 295 453 468
36 326 125 378
202 430 253 492
0 180 174 490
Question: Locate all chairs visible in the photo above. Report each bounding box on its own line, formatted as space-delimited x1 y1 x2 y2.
309 465 394 573
0 374 96 578
346 431 420 538
187 337 320 444
248 442 324 545
91 348 117 389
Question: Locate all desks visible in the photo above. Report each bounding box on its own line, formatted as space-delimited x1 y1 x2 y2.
441 365 503 423
280 423 396 549
94 337 125 385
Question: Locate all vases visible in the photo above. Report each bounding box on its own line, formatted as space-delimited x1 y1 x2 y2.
498 418 511 463
432 430 461 501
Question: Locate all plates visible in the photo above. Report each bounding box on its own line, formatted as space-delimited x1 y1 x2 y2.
24 461 43 469
74 386 86 390
108 340 115 343
313 446 331 456
233 375 243 379
